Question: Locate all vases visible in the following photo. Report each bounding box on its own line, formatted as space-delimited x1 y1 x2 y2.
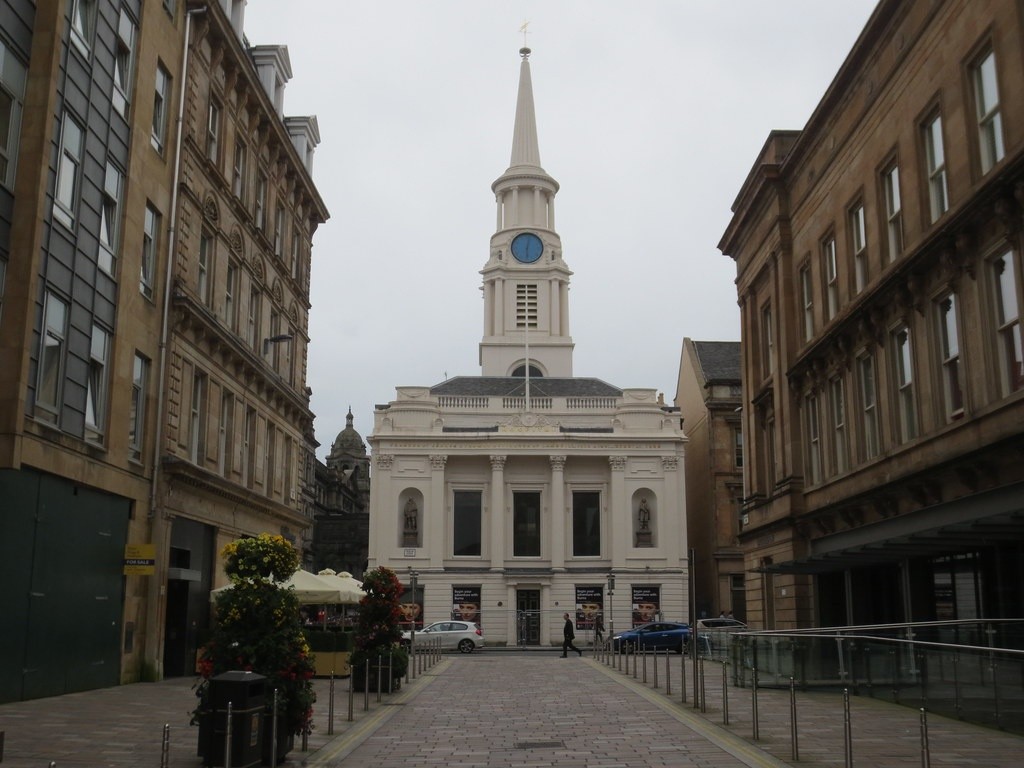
265 695 294 765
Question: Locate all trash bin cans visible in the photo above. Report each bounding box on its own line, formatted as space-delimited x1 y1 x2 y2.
201 671 270 768
255 660 294 765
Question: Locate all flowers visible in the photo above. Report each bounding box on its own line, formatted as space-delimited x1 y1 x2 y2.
344 565 404 647
186 533 316 736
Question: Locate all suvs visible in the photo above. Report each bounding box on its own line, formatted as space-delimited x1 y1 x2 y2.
687 616 748 651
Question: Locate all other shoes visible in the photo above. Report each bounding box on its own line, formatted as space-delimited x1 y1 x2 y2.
579 650 582 656
560 655 567 658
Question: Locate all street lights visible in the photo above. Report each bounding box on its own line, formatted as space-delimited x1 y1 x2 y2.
605 571 616 657
409 571 420 656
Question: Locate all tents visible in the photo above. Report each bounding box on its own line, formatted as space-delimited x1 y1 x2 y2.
209 564 374 632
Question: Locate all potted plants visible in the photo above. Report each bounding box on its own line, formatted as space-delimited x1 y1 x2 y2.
347 648 409 691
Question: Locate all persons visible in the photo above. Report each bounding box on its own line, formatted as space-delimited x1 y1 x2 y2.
560 613 582 658
404 496 417 530
719 610 734 620
638 496 650 530
596 616 605 644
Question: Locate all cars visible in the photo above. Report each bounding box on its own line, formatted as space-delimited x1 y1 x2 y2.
606 621 690 655
400 619 485 654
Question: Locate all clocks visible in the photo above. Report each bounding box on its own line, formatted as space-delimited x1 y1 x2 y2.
512 232 544 263
